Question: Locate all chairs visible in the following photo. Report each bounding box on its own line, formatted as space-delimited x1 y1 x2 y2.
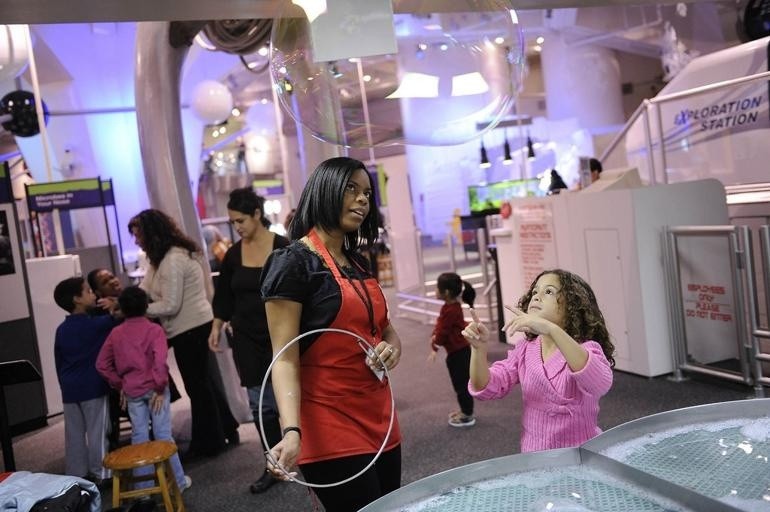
128 252 151 284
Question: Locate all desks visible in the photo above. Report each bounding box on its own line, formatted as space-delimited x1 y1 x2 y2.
458 213 496 262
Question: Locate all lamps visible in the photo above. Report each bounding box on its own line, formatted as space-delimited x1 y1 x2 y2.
475 44 538 169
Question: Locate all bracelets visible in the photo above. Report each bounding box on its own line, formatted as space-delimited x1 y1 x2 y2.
282 427 302 439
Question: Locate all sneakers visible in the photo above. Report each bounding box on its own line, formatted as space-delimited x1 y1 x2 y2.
170 476 192 497
200 430 240 459
448 410 475 427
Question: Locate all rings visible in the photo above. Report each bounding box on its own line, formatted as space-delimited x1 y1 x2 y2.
388 346 394 354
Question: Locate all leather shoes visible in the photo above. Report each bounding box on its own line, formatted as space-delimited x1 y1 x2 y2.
250 468 276 494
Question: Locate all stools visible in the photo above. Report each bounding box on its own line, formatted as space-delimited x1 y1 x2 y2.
0 471 87 512
99 407 185 512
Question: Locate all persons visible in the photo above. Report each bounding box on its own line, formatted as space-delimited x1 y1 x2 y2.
88 268 157 452
574 157 601 188
429 272 476 427
208 185 292 495
95 286 192 502
255 154 404 512
96 207 240 461
462 268 616 455
53 275 125 493
